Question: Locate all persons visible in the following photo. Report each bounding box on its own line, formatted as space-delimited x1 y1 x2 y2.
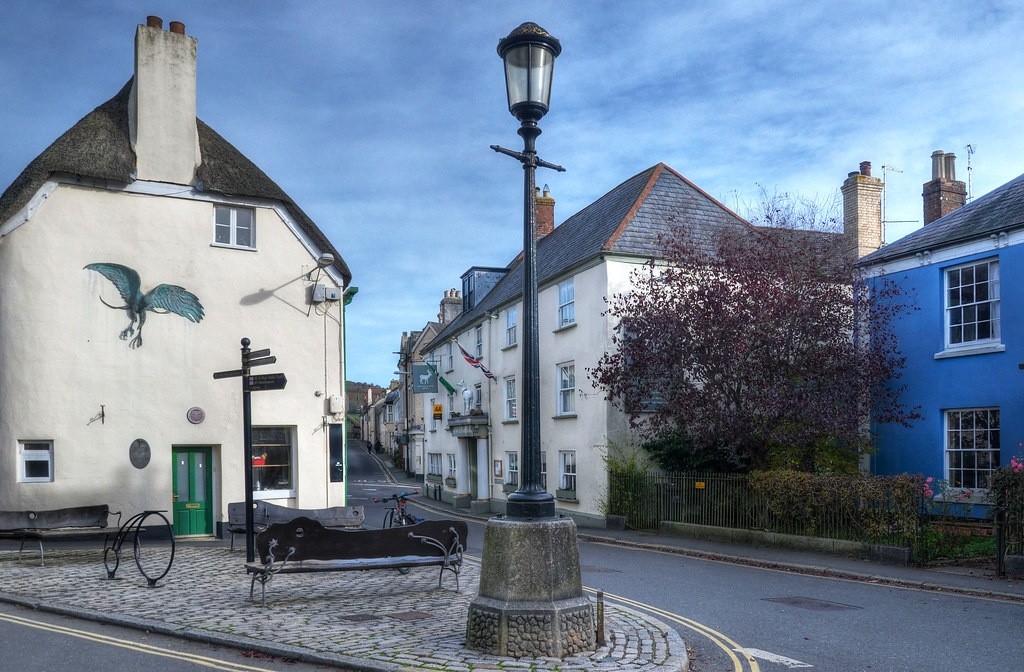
367 441 372 454
375 440 381 454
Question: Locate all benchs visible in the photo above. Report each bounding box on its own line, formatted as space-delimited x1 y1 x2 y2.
245 516 469 606
0 504 147 566
227 500 365 551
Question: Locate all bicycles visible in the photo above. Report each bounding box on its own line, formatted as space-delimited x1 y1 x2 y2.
373 487 424 576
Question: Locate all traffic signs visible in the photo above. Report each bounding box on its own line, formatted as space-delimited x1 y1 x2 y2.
247 356 276 369
212 368 250 380
246 373 287 392
245 348 270 360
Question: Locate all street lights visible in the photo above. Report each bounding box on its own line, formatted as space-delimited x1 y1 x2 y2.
461 21 608 661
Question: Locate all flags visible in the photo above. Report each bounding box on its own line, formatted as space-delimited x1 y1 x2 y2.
427 362 455 395
456 341 494 379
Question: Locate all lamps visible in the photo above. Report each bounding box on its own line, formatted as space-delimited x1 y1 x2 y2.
307 253 334 317
